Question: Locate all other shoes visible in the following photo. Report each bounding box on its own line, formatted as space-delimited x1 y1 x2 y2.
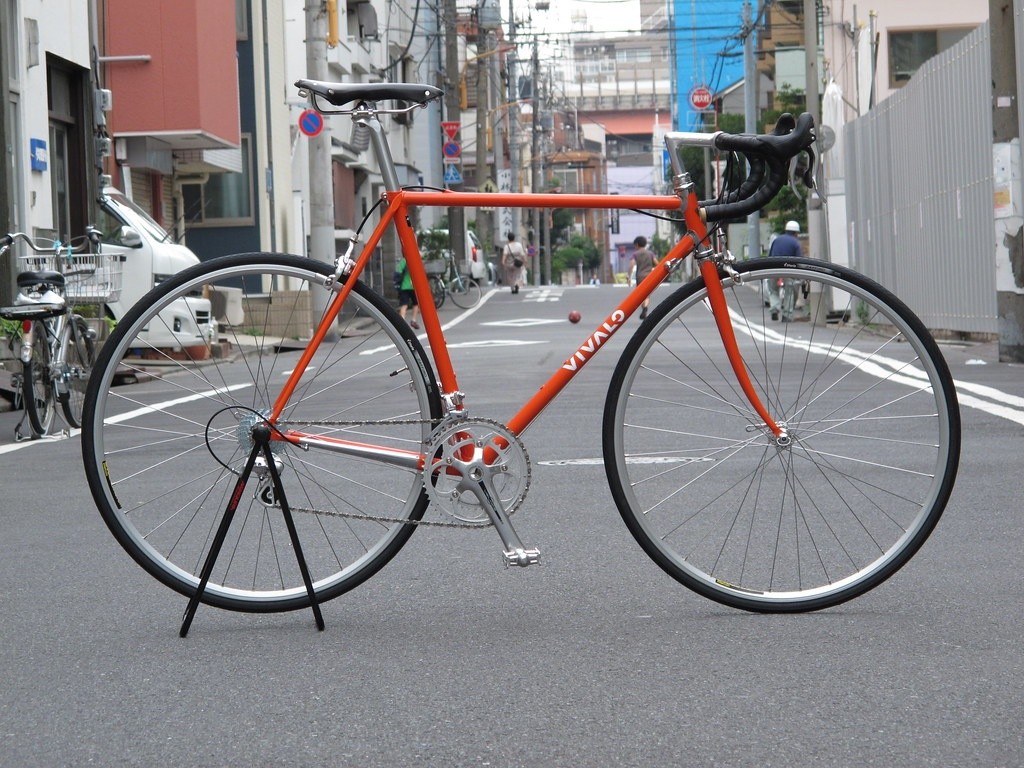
783 316 791 322
771 310 779 321
640 306 649 319
512 290 517 293
515 285 518 293
410 319 420 330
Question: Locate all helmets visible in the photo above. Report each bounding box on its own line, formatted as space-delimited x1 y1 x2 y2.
785 220 800 231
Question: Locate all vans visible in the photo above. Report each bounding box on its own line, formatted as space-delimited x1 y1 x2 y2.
423 228 484 284
96 175 214 361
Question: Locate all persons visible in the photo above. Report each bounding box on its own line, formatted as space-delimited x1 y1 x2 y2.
627 237 658 319
503 233 527 293
590 273 600 284
767 221 803 322
398 258 419 329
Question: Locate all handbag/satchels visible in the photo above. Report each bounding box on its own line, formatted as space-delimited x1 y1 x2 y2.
514 259 523 267
394 272 404 289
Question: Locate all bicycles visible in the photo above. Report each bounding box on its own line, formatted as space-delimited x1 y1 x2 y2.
426 251 482 310
0 225 126 443
81 79 961 637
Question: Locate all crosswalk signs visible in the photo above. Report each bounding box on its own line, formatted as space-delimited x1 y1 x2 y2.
443 163 464 184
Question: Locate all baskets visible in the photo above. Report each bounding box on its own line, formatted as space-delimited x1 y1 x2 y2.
18 253 126 303
458 259 473 277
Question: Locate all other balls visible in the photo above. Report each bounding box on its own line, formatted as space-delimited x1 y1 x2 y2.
568 310 581 323
